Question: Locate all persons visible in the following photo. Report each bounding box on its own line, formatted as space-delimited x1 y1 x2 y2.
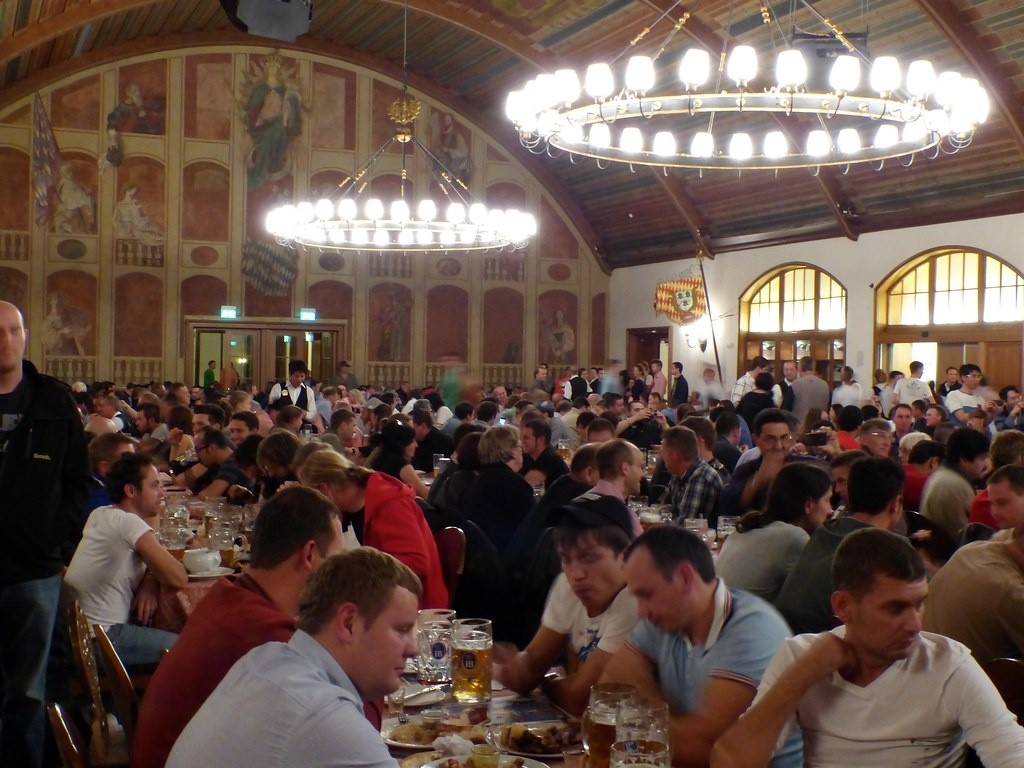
164 547 423 768
133 486 341 768
0 301 87 768
204 360 216 388
64 352 1023 661
587 524 804 768
710 527 1024 768
269 360 316 432
329 361 359 390
230 362 240 388
489 500 641 717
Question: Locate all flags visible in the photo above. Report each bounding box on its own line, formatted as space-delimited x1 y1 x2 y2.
654 277 707 326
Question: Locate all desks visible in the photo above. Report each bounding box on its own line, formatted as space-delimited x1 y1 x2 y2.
154 464 588 768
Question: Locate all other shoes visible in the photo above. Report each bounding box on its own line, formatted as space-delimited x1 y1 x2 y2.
47 700 91 768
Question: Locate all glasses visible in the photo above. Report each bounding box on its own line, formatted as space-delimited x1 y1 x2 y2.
966 373 983 380
194 444 209 455
757 433 793 444
865 429 896 443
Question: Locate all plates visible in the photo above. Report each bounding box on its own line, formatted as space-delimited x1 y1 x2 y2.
486 720 585 757
394 685 445 706
380 715 434 749
188 566 235 578
419 477 434 485
420 753 550 768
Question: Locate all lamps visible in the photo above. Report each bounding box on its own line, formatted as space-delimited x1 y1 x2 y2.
506 0 992 180
682 326 708 353
265 0 537 256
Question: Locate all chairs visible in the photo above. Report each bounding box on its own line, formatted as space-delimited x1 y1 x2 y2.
46 601 151 768
965 657 1024 768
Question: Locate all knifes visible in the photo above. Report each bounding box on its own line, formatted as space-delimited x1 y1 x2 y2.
396 683 449 700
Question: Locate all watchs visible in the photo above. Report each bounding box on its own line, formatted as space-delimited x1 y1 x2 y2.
544 672 560 682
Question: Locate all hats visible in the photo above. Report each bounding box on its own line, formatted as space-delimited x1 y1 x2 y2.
336 361 351 369
543 493 632 543
535 399 554 418
408 399 433 415
361 397 389 410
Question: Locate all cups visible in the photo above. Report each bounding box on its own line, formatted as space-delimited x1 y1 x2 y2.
203 495 263 567
433 453 451 478
152 492 192 562
638 447 662 479
405 608 493 705
528 481 545 502
557 438 571 460
627 494 741 557
581 680 673 768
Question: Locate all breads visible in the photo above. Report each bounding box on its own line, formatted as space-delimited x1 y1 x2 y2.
434 712 485 743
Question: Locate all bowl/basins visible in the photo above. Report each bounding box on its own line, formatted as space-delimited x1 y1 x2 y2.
182 548 222 574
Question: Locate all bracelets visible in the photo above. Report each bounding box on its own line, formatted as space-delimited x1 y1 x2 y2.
627 418 632 424
